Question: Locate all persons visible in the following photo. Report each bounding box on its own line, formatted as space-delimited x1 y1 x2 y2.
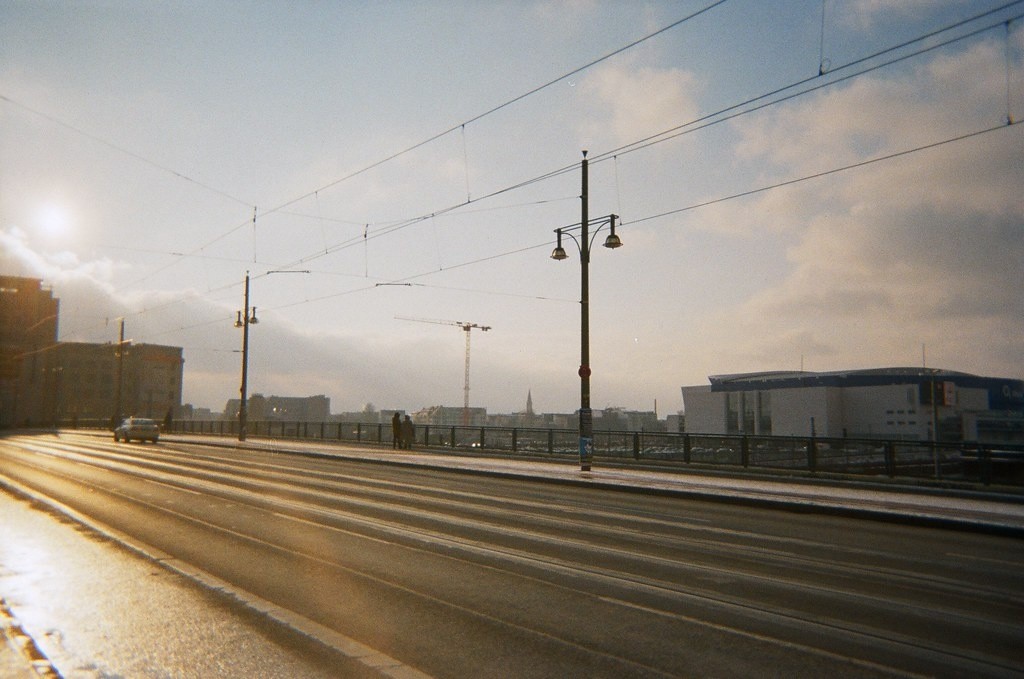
163 407 174 433
398 416 415 450
391 412 402 450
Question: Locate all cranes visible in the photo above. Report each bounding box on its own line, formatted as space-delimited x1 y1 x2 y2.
394 314 494 426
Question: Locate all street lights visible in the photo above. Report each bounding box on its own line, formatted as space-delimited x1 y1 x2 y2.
232 272 264 443
551 152 624 470
112 318 133 428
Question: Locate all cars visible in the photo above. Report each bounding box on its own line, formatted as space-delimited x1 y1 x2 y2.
114 417 159 445
